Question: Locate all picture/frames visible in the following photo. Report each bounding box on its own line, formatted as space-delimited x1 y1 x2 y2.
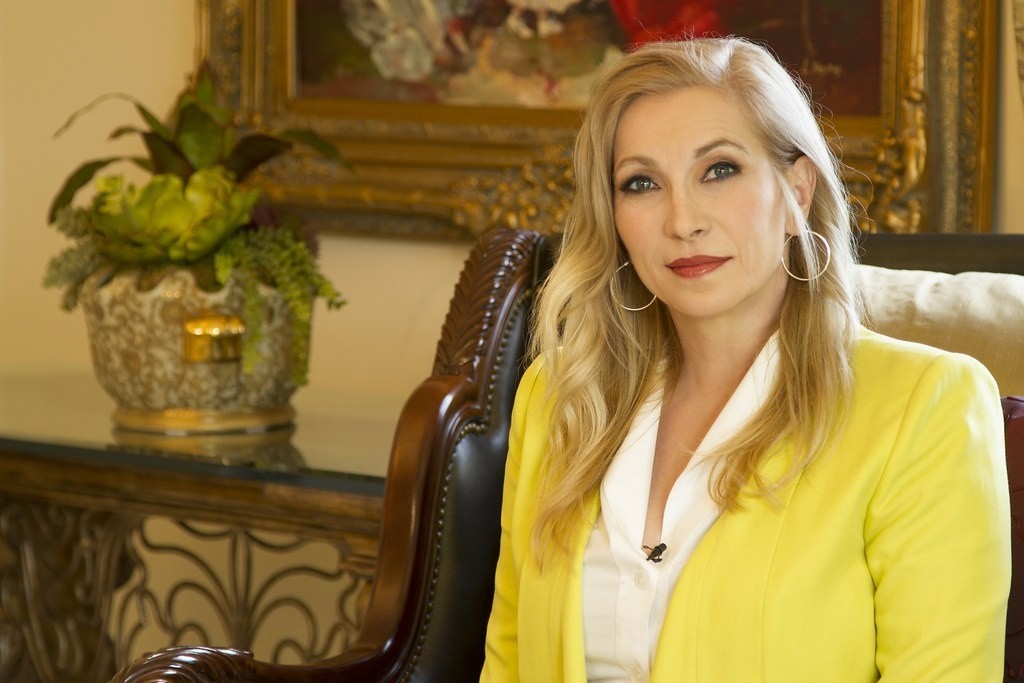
192 0 1003 245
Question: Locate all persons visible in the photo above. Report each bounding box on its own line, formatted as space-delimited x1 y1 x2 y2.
480 38 1012 683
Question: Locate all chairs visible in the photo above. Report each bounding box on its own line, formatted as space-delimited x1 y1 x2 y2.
108 223 1024 683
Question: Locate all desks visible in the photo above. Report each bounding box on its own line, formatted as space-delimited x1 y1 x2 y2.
0 367 394 683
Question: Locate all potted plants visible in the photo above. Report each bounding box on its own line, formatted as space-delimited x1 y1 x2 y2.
42 54 359 438
